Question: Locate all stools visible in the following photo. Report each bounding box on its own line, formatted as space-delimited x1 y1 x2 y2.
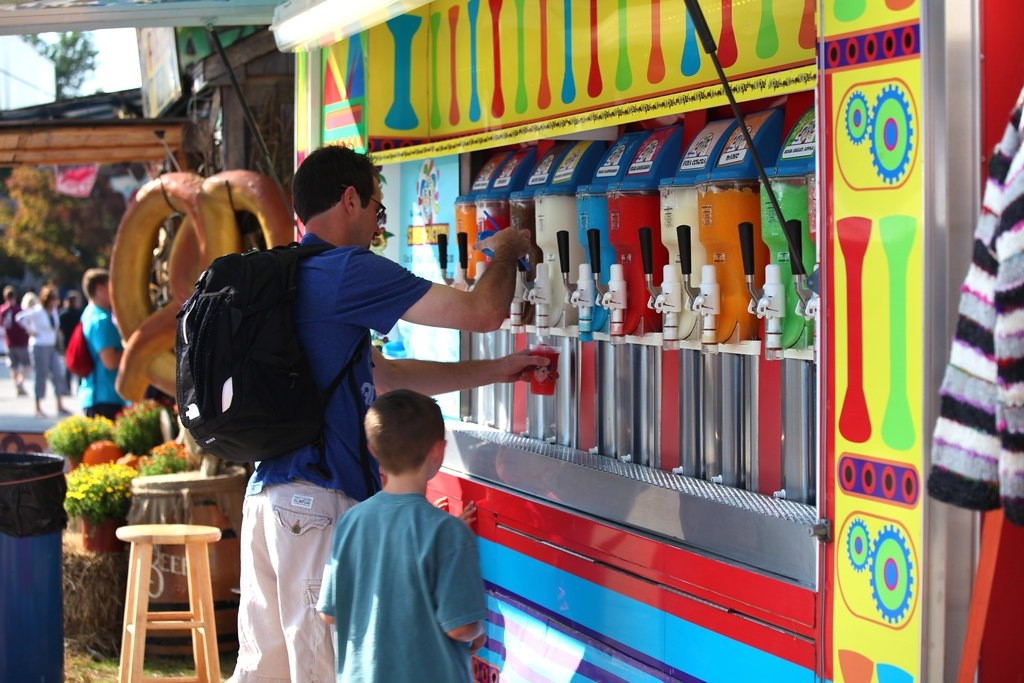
116 522 223 682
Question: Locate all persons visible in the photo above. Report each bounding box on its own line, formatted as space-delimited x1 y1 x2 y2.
220 145 548 682
0 281 83 420
77 268 123 424
431 496 477 530
314 388 490 682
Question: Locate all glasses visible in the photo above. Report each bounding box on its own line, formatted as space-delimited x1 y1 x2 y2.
342 184 386 221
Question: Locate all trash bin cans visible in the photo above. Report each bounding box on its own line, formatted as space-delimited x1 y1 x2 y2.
0 450 68 683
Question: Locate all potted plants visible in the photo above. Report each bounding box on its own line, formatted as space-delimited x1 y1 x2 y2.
45 397 199 555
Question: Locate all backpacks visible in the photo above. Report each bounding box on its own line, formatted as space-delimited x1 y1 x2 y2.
175 241 335 462
66 322 94 376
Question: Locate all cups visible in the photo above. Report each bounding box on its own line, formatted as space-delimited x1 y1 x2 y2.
531 348 560 395
